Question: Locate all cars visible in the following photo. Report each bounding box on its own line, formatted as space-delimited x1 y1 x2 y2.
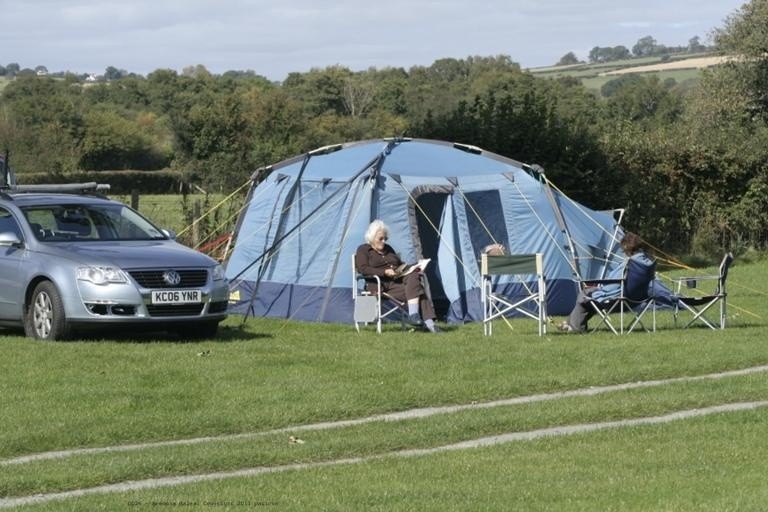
0 180 229 344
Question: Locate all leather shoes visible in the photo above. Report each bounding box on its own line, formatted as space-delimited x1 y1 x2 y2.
423 323 444 332
407 313 422 326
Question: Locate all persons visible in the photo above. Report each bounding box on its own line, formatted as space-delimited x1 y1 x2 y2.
353 216 445 336
553 231 651 335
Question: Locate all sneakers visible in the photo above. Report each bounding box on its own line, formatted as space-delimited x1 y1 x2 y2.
555 320 568 331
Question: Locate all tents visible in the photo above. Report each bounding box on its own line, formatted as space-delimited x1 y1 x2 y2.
221 135 687 325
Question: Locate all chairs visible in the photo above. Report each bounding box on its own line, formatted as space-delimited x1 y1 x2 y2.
671 252 734 330
481 253 547 336
352 254 433 333
58 217 91 239
583 257 658 335
